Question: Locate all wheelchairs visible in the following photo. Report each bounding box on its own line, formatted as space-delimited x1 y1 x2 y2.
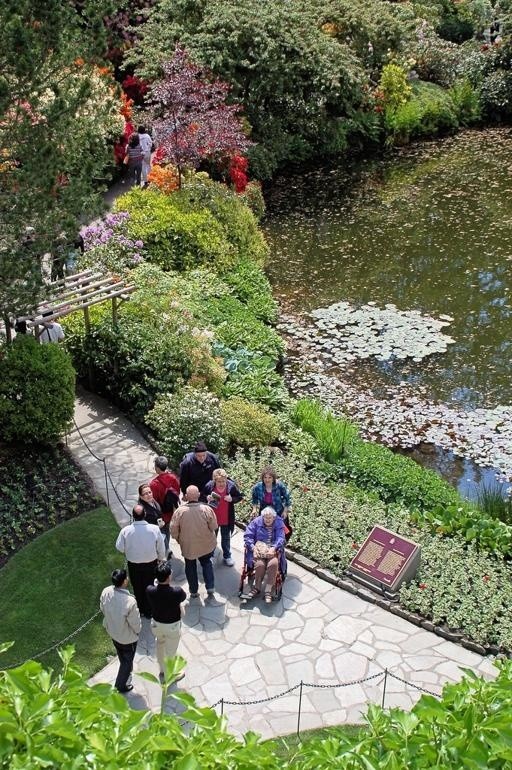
234 545 284 604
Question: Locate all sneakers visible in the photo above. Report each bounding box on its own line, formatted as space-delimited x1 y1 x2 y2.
265 592 271 602
248 589 258 597
224 558 234 565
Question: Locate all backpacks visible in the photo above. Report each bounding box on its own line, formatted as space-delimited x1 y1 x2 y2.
156 478 180 513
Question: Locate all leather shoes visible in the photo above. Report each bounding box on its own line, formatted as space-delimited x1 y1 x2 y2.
118 685 133 692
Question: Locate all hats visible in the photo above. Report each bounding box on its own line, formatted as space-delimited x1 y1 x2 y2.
195 442 207 452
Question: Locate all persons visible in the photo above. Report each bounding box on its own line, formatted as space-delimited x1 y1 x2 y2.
100 568 142 692
145 562 187 678
1 124 153 347
115 442 291 618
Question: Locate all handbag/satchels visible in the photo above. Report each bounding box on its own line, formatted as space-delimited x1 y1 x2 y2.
253 540 274 559
